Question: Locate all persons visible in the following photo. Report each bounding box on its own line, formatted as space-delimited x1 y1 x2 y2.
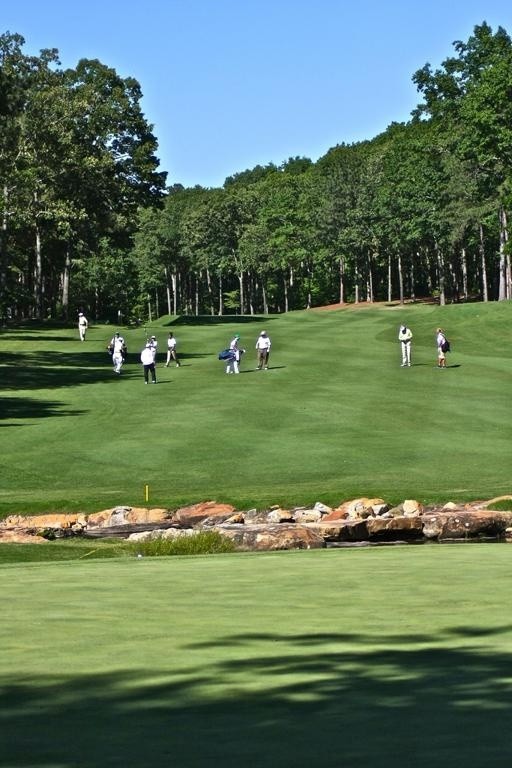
146 335 158 367
435 328 450 367
226 335 246 374
140 344 156 383
163 331 180 368
77 313 88 341
109 330 125 374
397 324 413 367
255 330 271 370
